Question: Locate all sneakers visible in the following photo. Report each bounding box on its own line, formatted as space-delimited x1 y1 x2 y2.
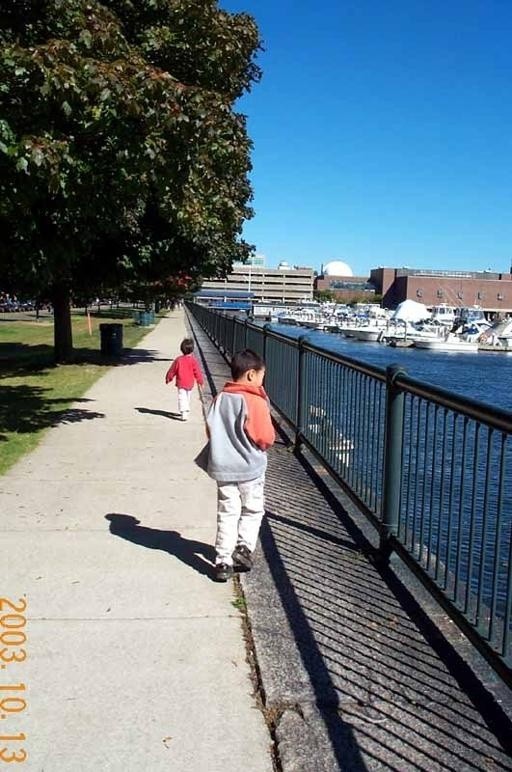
182 411 191 420
232 545 254 571
216 562 233 579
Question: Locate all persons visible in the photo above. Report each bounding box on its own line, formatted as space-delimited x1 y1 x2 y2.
205 350 277 580
165 339 205 421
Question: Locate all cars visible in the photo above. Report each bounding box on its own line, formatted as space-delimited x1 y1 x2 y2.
92 297 146 308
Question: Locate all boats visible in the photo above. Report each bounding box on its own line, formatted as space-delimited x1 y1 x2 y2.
277 299 512 352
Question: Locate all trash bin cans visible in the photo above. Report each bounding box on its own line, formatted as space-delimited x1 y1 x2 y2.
99 323 123 357
133 311 155 326
